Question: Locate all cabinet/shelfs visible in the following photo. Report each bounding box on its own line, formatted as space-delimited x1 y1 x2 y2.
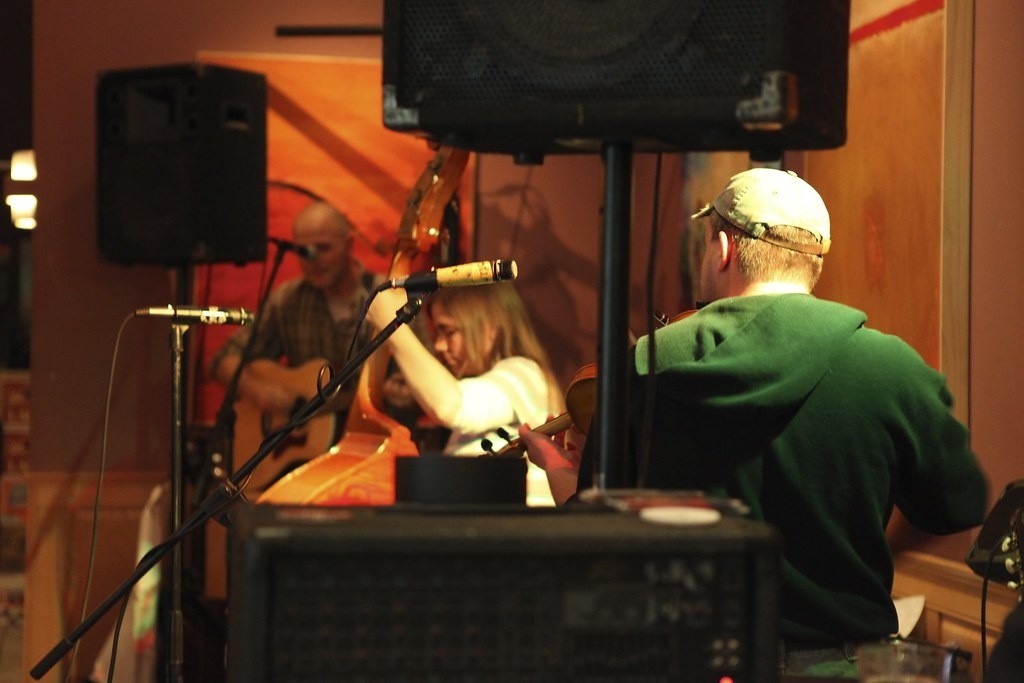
219 502 788 682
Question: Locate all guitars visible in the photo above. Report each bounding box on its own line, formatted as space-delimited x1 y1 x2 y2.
231 356 404 493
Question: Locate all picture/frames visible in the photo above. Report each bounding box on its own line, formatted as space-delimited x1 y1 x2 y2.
184 50 478 604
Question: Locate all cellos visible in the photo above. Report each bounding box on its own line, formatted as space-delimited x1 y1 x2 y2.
256 140 474 509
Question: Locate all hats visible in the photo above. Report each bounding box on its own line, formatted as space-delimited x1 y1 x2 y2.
692 167 831 254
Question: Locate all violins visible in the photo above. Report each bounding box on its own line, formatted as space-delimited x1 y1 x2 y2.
479 307 707 461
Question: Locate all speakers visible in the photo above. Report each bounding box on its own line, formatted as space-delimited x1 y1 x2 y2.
380 0 850 164
94 62 265 265
234 503 780 682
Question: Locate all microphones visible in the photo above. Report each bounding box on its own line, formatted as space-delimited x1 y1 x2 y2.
389 258 520 290
270 236 319 262
136 305 256 326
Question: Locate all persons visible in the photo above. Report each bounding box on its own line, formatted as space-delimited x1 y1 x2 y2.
210 201 438 510
573 165 991 679
363 261 570 507
